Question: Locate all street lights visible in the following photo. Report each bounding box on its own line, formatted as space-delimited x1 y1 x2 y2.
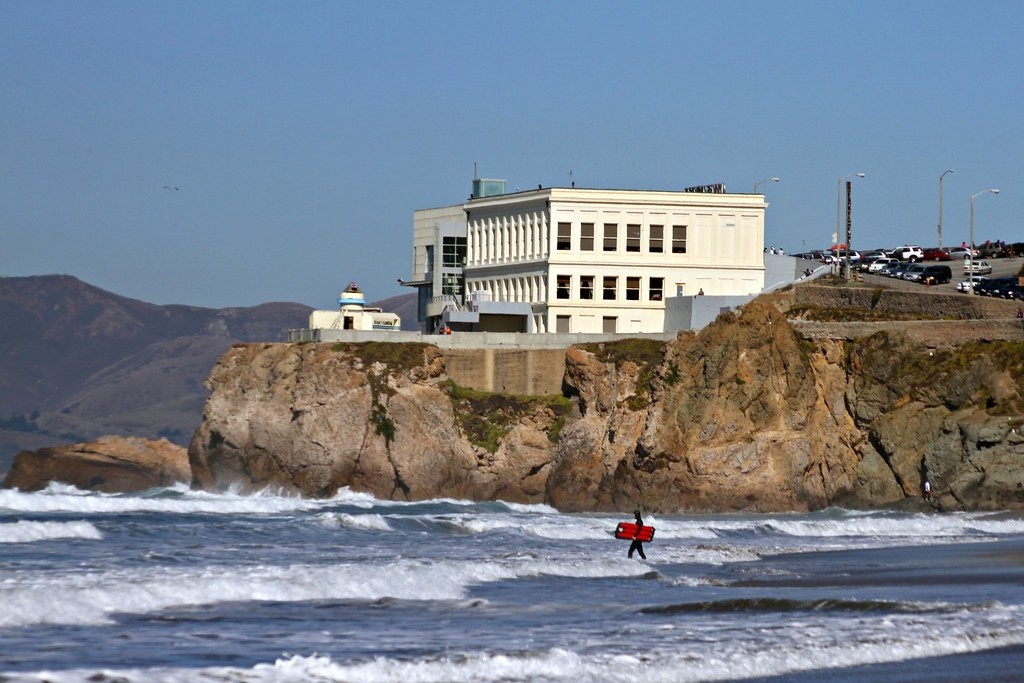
754 177 780 193
939 170 954 248
836 172 865 277
968 188 1000 295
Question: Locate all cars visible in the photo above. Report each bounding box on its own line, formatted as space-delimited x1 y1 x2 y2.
963 261 992 275
977 242 1004 259
804 249 952 285
1011 242 1024 257
922 248 951 262
956 275 1024 301
948 246 982 260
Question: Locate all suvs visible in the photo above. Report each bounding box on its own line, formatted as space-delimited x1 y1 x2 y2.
885 245 924 263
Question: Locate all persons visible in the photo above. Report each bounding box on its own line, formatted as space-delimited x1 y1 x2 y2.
800 269 813 277
445 326 450 335
439 325 444 334
925 272 930 287
997 240 1005 250
764 245 784 255
830 258 846 267
962 242 966 248
698 288 704 296
628 510 646 559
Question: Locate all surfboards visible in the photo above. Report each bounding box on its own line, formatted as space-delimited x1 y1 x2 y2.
614 522 656 542
